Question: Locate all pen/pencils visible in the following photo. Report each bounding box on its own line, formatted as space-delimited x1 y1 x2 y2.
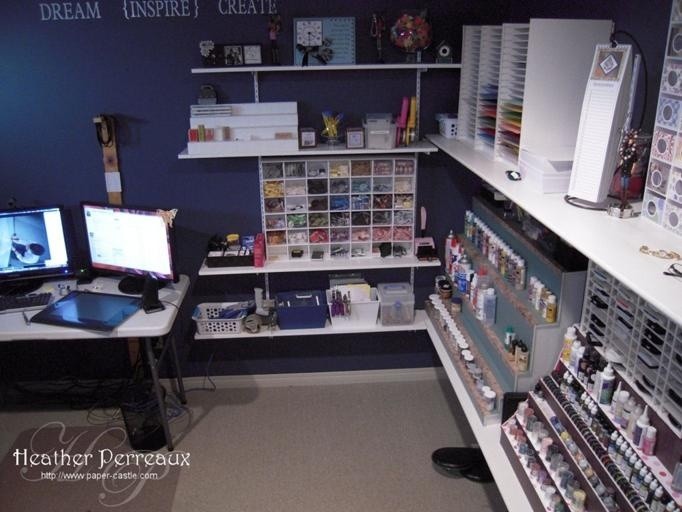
22 311 31 326
57 284 70 289
331 289 351 317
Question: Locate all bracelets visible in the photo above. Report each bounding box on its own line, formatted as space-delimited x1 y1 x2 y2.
14 249 17 253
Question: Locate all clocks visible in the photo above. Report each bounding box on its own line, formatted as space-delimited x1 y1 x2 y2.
296 20 323 47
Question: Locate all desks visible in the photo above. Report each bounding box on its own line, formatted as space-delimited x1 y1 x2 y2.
1 275 193 449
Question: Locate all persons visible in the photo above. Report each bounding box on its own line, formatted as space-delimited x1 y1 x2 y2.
10 234 47 267
619 138 637 208
265 13 282 65
226 47 241 64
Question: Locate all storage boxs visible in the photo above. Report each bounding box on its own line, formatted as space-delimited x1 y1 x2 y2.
324 287 382 329
358 120 398 151
275 289 329 329
376 281 416 327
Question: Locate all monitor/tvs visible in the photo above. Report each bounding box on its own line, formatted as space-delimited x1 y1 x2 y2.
79 201 180 294
0 206 76 297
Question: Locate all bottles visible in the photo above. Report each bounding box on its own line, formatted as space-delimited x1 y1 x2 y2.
435 203 681 511
186 125 228 141
207 234 254 257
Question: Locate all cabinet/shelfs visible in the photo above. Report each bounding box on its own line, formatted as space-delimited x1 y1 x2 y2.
500 260 682 511
419 125 682 511
422 191 590 427
172 61 448 339
640 2 681 238
455 18 613 170
184 102 299 156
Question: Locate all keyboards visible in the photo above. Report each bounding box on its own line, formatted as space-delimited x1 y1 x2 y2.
0 292 53 315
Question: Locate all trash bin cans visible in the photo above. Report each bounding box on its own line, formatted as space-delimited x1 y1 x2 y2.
118 382 167 453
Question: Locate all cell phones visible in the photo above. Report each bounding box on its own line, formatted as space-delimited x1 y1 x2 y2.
143 279 158 306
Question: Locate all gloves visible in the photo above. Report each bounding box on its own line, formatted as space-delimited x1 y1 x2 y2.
191 300 250 334
